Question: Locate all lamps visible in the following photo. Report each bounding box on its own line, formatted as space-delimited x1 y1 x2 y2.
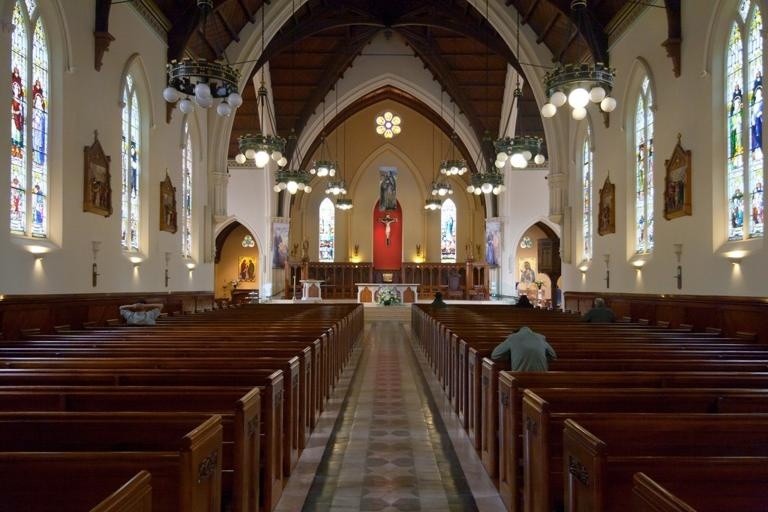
352 239 361 263
414 243 424 264
728 256 742 264
163 0 615 211
133 262 142 267
33 252 44 260
520 232 534 249
241 235 255 247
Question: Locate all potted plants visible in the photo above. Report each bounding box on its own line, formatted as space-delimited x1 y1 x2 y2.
447 268 462 290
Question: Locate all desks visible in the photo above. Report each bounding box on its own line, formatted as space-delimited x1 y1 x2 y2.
299 279 326 300
355 282 420 304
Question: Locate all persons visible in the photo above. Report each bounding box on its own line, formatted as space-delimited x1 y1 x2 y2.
515 294 534 308
243 265 248 272
583 298 617 323
491 326 559 372
247 259 254 279
522 261 535 282
117 299 165 326
380 171 397 211
430 291 446 305
381 216 396 239
241 259 248 279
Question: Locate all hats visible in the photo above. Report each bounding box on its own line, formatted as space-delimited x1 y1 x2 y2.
434 292 444 298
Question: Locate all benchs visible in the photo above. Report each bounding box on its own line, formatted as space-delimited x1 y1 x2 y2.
1 300 364 512
409 302 768 511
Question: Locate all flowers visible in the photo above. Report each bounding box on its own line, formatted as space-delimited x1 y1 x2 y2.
374 286 402 306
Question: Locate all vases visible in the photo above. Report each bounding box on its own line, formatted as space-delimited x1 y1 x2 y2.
384 301 391 306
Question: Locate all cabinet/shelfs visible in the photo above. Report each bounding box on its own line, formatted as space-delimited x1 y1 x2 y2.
537 239 561 274
231 289 258 304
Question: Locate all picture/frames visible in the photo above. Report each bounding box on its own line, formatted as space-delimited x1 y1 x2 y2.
160 169 177 233
598 171 616 236
83 129 113 218
664 132 691 220
